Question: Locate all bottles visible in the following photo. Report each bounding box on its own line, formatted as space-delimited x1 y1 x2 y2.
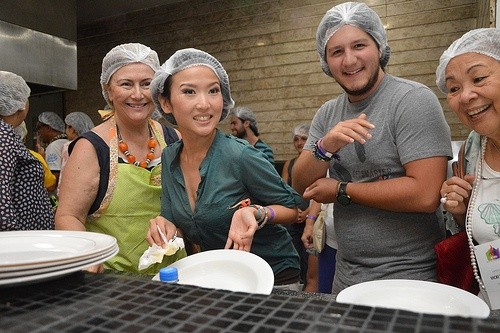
158 267 178 285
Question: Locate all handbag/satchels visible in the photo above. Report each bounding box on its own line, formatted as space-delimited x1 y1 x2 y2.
313 211 327 253
434 141 481 296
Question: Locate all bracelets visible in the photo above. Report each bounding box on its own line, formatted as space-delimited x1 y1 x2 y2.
305 214 317 221
335 181 341 197
249 205 275 230
311 139 341 162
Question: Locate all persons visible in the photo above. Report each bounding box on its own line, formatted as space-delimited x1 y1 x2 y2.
290 2 454 293
304 254 320 293
1 70 97 233
53 42 187 275
436 27 500 311
230 106 275 169
301 168 339 294
281 123 310 289
145 48 302 293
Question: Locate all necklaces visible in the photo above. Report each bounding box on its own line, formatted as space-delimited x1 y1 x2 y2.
45 134 67 146
115 124 156 168
467 135 487 289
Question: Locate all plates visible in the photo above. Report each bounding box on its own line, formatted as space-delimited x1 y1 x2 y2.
335 279 491 319
151 248 274 296
0 230 120 285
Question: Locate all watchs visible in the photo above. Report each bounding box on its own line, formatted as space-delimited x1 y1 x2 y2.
336 181 354 206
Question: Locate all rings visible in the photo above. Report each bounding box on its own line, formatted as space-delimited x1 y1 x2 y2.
440 193 449 203
